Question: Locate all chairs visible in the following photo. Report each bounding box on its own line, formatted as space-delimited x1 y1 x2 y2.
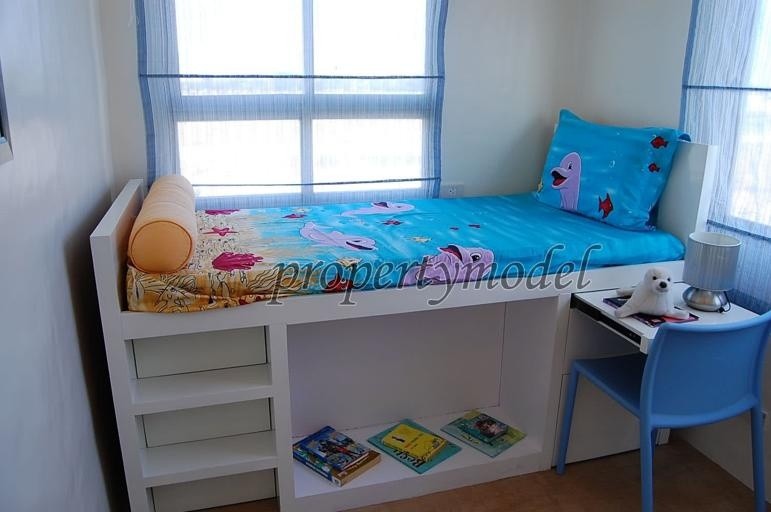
555 309 771 512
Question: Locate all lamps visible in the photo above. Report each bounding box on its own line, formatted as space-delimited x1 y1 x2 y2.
683 231 741 312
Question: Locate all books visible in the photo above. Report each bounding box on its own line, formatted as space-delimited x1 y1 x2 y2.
607 293 700 328
442 408 529 461
365 417 463 476
383 423 448 462
456 411 510 444
292 424 383 488
301 427 370 468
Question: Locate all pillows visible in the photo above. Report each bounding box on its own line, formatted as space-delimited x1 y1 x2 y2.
533 109 692 233
126 175 197 274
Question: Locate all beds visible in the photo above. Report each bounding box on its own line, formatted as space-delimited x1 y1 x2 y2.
89 123 721 511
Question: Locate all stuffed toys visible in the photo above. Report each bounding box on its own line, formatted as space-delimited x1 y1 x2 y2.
613 264 692 322
